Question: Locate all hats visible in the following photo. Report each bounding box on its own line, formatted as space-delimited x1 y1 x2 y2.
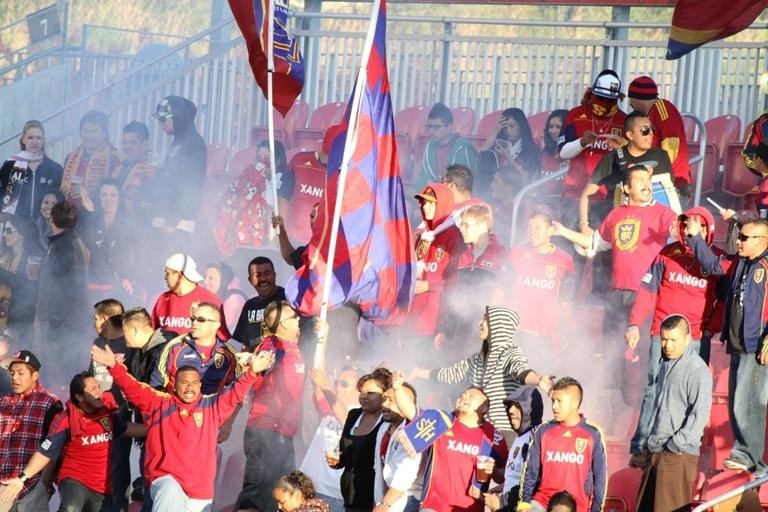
592 74 621 100
165 254 203 282
414 188 436 202
149 99 172 121
628 76 658 100
684 215 706 226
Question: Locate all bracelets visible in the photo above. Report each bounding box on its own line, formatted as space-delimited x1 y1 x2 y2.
578 221 589 229
18 472 30 484
381 502 391 508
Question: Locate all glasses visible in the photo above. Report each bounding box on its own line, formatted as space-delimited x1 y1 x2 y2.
4 228 15 234
287 314 299 319
190 315 215 322
641 125 655 135
426 124 444 129
334 380 350 388
737 233 765 241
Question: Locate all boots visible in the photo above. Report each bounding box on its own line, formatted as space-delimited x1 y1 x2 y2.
11 350 40 371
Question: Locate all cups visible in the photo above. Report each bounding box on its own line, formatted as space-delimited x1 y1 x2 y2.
25 254 43 284
476 454 490 483
326 437 340 464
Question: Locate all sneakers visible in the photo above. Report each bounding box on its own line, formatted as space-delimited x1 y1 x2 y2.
725 457 747 473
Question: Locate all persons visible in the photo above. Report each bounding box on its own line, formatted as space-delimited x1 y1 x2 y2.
545 489 577 512
391 370 510 512
622 206 730 468
236 497 259 512
324 365 392 512
298 366 362 511
514 376 609 512
740 112 768 179
602 75 692 201
81 177 138 298
681 214 768 471
575 110 677 311
549 164 679 382
439 163 492 229
57 109 119 212
142 95 208 250
478 107 541 185
430 204 512 399
1 215 48 351
276 125 339 244
233 297 331 512
399 303 556 433
0 120 63 253
88 342 276 512
1 322 34 394
232 255 287 352
489 165 553 244
0 369 148 512
555 69 629 297
483 382 544 512
147 302 237 395
402 182 466 339
627 313 715 512
120 306 180 478
36 199 92 385
270 202 363 371
254 139 296 222
272 470 331 512
87 298 135 511
32 189 64 251
537 109 569 194
718 142 768 226
0 348 66 512
150 253 233 343
371 381 429 512
202 260 250 335
109 119 159 211
409 101 478 224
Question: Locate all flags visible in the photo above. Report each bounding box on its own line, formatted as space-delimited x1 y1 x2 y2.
283 1 418 343
227 0 307 118
665 0 768 63
